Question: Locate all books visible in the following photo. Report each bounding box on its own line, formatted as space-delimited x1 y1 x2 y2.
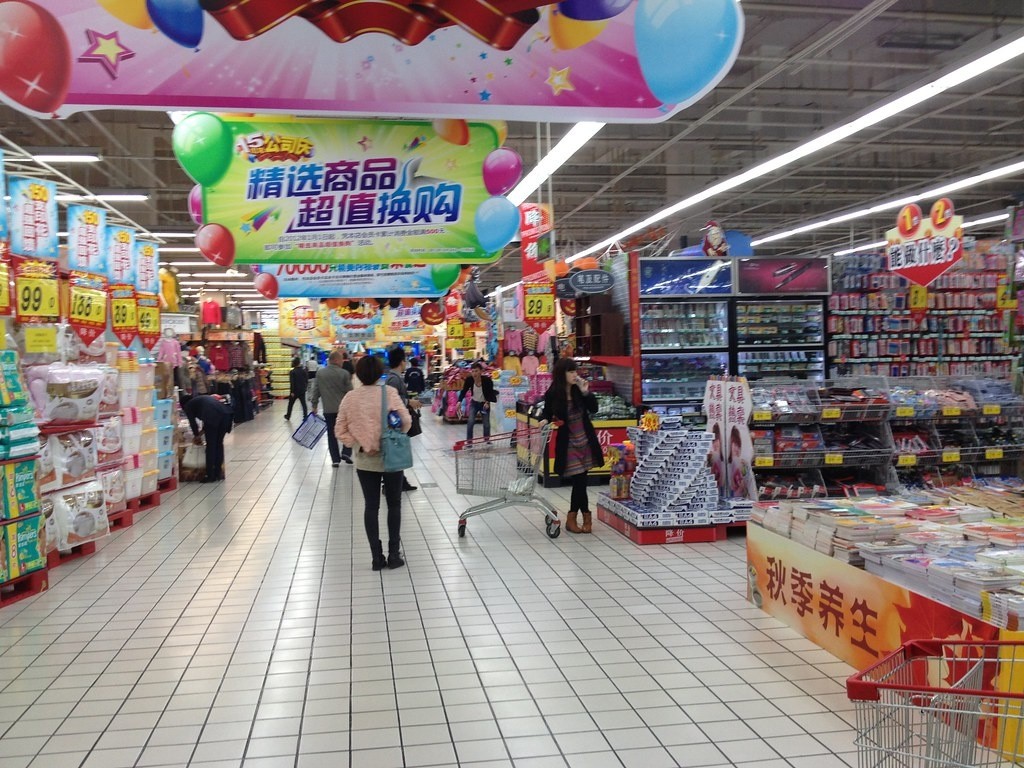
750 486 1024 630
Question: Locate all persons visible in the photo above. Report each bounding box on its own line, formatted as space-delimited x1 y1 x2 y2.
179 395 234 484
334 355 412 571
710 423 726 496
157 327 183 370
284 357 308 421
382 347 422 494
195 338 249 375
404 358 425 393
726 425 748 498
457 362 497 447
311 350 354 467
538 358 604 533
306 357 318 378
342 352 355 374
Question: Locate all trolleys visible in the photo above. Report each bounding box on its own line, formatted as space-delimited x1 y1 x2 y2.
847 637 1024 767
452 419 561 538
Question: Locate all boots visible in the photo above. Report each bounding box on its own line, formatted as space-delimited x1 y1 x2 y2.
370 540 388 571
582 511 592 533
566 512 582 533
388 539 404 568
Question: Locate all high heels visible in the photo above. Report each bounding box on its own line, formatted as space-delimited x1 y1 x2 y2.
201 472 221 483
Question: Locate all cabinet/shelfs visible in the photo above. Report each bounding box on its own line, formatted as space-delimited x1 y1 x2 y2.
739 371 1024 513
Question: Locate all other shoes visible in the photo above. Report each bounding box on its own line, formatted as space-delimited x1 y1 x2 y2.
402 484 417 491
332 462 339 467
342 454 353 464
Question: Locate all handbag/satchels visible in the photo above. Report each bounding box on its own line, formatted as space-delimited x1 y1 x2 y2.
389 403 423 437
382 431 413 471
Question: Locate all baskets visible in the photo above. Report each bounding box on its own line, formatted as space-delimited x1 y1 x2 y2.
292 412 328 450
